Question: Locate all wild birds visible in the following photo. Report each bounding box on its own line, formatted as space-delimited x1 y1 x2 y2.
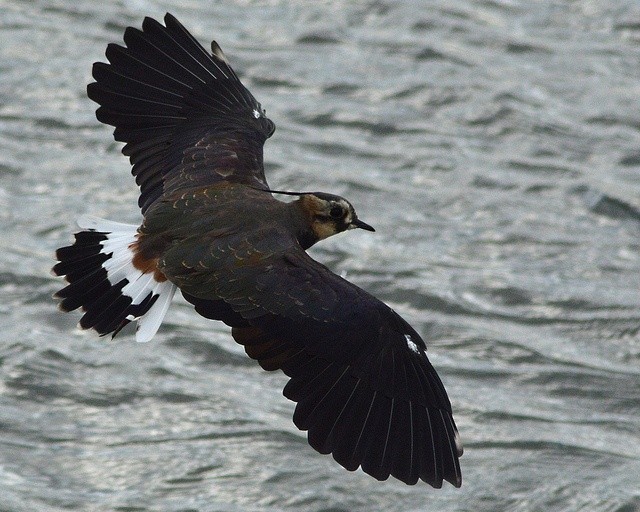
48 12 464 491
